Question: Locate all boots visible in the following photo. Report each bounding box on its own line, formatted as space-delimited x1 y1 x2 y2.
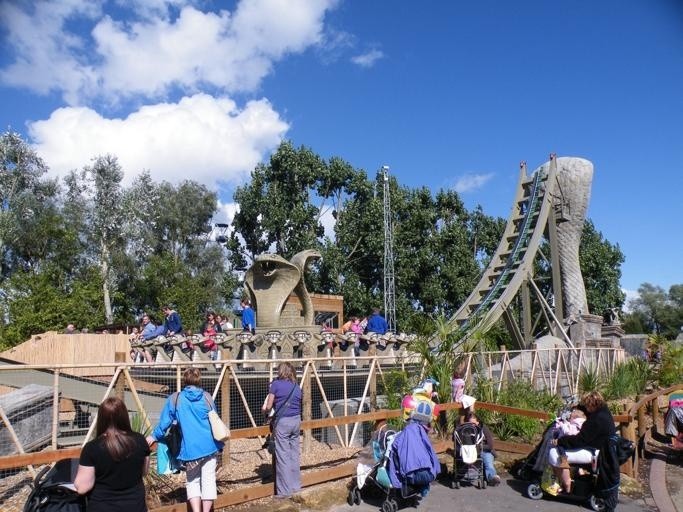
559 456 570 469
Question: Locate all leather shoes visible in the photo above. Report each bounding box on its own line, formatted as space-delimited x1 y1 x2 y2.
566 479 575 496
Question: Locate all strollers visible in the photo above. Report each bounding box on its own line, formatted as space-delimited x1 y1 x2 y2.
522 418 634 511
23 458 90 512
347 421 437 511
451 423 490 490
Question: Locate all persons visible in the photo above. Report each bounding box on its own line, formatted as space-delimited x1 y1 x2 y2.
67 324 138 335
146 366 225 512
73 396 150 511
645 350 662 362
196 311 234 360
265 362 303 500
320 300 387 357
445 356 621 512
133 306 183 363
232 300 255 361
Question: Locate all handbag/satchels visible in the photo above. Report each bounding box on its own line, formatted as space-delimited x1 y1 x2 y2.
615 438 636 465
204 394 230 443
459 445 477 465
165 392 181 470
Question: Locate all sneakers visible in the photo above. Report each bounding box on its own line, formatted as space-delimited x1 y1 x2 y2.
486 475 500 486
